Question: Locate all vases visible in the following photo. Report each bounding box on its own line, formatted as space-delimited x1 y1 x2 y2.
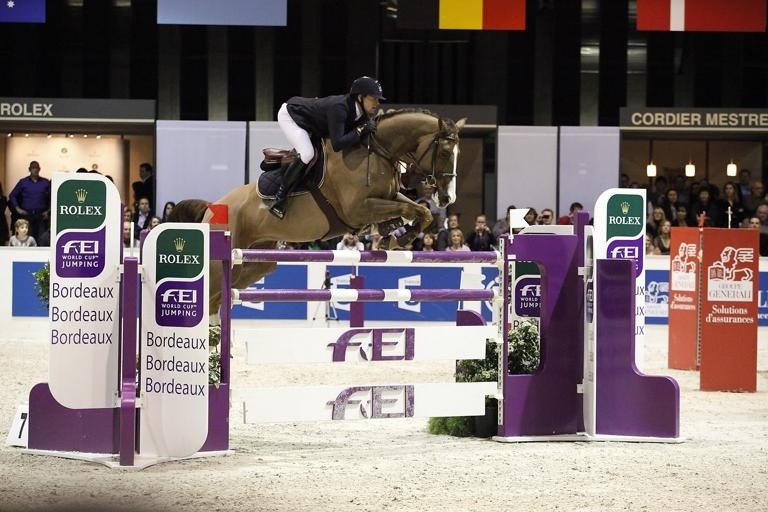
473 407 498 437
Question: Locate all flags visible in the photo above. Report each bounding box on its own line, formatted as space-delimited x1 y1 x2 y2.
438 0 527 31
0 0 46 24
635 0 767 34
155 0 288 27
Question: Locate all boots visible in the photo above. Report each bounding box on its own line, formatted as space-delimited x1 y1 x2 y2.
271 157 308 219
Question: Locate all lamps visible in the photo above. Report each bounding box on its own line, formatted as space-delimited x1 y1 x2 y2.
646 160 657 177
726 158 736 176
684 158 695 177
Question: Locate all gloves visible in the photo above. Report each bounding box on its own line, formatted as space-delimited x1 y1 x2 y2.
359 120 377 139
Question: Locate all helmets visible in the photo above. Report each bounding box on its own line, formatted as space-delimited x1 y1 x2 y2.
350 76 387 100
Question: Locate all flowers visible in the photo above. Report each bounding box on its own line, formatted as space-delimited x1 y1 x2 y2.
457 319 540 407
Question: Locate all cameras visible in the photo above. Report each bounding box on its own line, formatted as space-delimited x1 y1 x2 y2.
542 215 549 219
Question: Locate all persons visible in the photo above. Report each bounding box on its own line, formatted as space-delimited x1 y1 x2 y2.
3 162 50 248
525 202 583 225
621 168 768 255
77 163 176 245
267 75 388 219
303 200 517 252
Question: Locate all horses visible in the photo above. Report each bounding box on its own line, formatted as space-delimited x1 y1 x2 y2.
165 107 469 326
